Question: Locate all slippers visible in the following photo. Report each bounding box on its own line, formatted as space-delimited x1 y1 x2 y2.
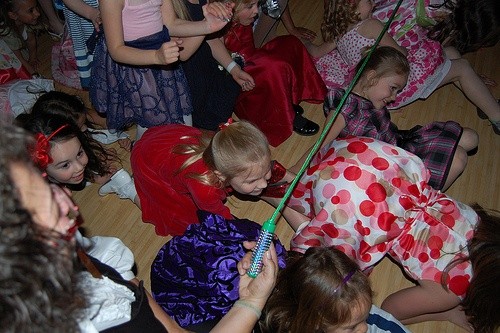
47 26 63 38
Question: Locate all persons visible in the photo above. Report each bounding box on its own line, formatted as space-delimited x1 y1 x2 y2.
285 46 478 202
97 118 298 238
316 0 500 134
139 209 409 333
0 143 280 333
258 134 500 333
0 0 328 195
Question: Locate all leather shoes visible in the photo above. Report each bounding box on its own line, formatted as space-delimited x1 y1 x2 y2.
292 114 320 137
291 104 304 115
98 167 132 196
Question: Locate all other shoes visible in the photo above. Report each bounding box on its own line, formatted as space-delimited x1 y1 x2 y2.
267 159 286 184
259 182 287 199
